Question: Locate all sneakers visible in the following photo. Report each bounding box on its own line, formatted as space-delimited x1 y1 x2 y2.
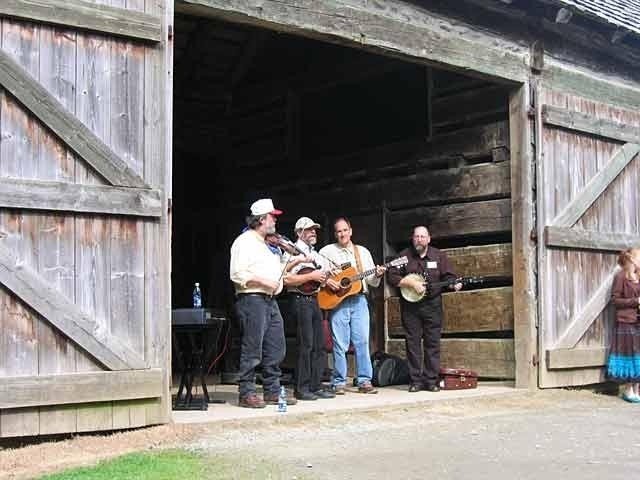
315 392 335 398
239 396 265 407
264 392 296 405
294 393 316 400
359 385 377 394
335 386 345 394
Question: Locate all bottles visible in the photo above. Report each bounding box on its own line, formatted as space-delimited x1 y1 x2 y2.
192 282 202 308
278 385 287 412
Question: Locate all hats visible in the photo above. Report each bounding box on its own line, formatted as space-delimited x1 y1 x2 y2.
294 217 321 232
250 199 283 215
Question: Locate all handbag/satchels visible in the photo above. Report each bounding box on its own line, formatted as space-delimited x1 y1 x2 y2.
371 351 410 387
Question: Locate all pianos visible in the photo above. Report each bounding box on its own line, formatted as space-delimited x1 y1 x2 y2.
172 308 228 326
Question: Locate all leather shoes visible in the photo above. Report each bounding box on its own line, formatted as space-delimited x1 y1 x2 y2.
425 384 439 392
409 385 424 392
623 393 639 402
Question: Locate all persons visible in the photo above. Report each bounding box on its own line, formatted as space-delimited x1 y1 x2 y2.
318 217 388 396
229 197 298 408
386 224 464 393
280 216 344 402
602 248 640 404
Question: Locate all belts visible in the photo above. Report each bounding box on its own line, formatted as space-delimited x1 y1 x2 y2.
289 293 313 299
238 292 266 297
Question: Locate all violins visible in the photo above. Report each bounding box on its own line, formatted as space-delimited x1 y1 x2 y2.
266 233 321 270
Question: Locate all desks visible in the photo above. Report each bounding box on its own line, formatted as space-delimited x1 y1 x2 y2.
171 324 224 410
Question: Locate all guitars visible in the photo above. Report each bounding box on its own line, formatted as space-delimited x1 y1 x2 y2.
316 255 408 310
